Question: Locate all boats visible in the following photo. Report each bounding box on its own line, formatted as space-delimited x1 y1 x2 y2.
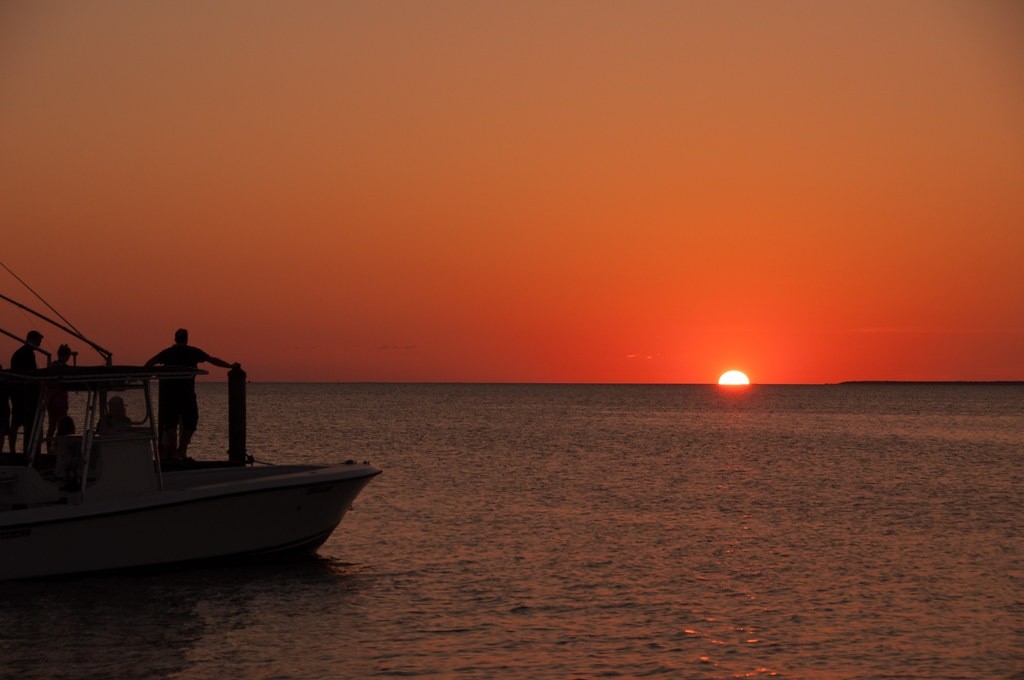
0 263 385 577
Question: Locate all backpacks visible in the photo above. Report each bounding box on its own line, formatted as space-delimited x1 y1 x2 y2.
43 464 82 504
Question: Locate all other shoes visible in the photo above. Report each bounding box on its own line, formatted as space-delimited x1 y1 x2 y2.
178 457 196 462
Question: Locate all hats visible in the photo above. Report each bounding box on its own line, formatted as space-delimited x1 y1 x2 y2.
108 397 128 408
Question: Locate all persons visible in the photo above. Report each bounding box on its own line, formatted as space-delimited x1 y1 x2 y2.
8 331 44 453
0 365 11 452
145 329 241 462
50 393 131 471
44 344 72 444
